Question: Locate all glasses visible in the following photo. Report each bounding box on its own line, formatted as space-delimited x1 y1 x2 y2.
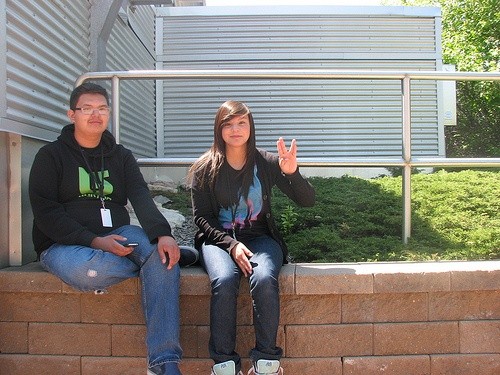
73 105 110 115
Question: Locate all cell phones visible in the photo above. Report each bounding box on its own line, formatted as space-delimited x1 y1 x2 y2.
125 244 139 247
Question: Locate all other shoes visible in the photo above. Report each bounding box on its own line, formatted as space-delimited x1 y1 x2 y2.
248 360 284 375
211 361 244 375
177 245 199 267
146 360 182 375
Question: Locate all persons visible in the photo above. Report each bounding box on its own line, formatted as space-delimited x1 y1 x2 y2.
29 83 199 375
184 100 314 375
